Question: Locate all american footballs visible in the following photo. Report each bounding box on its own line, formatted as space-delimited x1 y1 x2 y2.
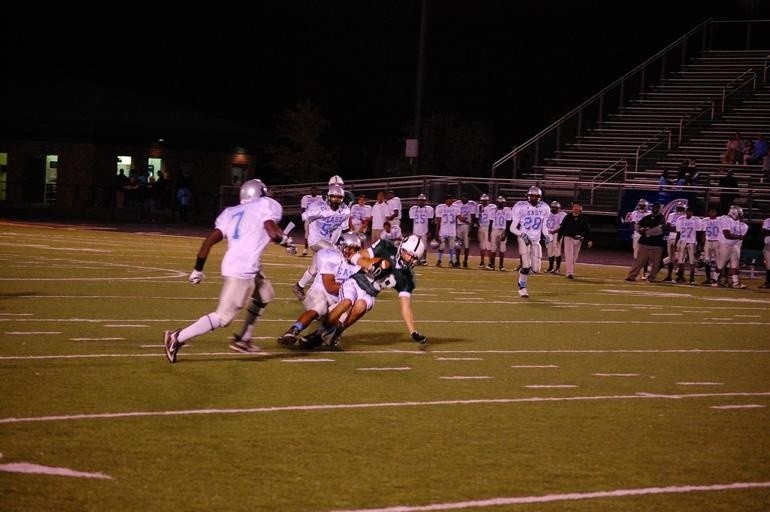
368 257 391 270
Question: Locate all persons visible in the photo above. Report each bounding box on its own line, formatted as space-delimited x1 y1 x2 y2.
509 185 591 297
282 225 429 353
162 179 298 363
94 164 240 223
287 174 405 247
621 135 770 292
408 193 512 272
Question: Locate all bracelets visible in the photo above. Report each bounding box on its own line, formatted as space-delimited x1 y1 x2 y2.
194 251 207 271
280 233 288 248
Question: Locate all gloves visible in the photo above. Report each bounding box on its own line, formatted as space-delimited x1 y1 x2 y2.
189 269 205 286
413 332 429 346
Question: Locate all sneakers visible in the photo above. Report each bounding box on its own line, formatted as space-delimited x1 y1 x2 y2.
518 288 529 297
662 278 746 288
229 338 259 353
544 269 560 274
277 327 341 349
642 272 648 280
437 260 505 270
165 330 184 363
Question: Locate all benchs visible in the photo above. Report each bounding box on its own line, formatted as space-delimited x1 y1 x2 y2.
489 49 770 222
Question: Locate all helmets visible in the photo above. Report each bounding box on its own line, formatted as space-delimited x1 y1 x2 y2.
328 175 344 209
528 186 542 196
675 202 687 214
400 235 425 268
341 231 361 248
550 201 561 208
638 199 648 211
240 179 267 199
729 205 744 219
480 193 506 202
